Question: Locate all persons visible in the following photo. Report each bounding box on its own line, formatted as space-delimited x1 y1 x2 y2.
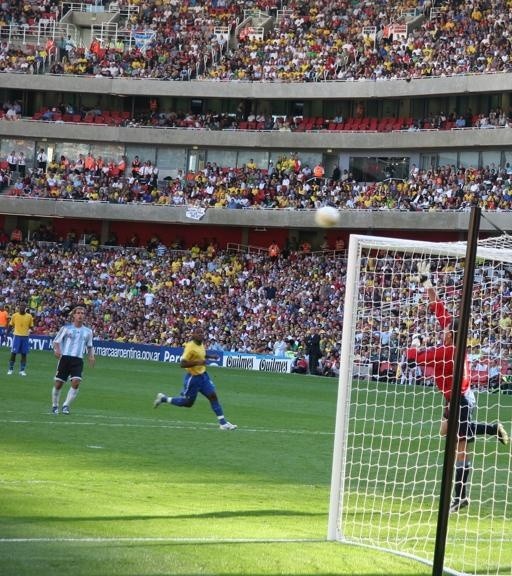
52 306 95 414
1 234 512 388
1 102 511 214
2 1 511 84
403 262 508 513
154 326 237 430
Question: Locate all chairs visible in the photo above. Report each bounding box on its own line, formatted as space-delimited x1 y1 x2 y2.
31 106 130 127
239 117 456 131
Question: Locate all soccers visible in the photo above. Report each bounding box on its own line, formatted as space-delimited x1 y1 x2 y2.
315 206 340 228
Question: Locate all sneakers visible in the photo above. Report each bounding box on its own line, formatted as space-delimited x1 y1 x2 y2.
153 393 165 409
491 423 508 445
7 369 27 377
220 421 237 430
52 403 70 415
451 496 468 512
